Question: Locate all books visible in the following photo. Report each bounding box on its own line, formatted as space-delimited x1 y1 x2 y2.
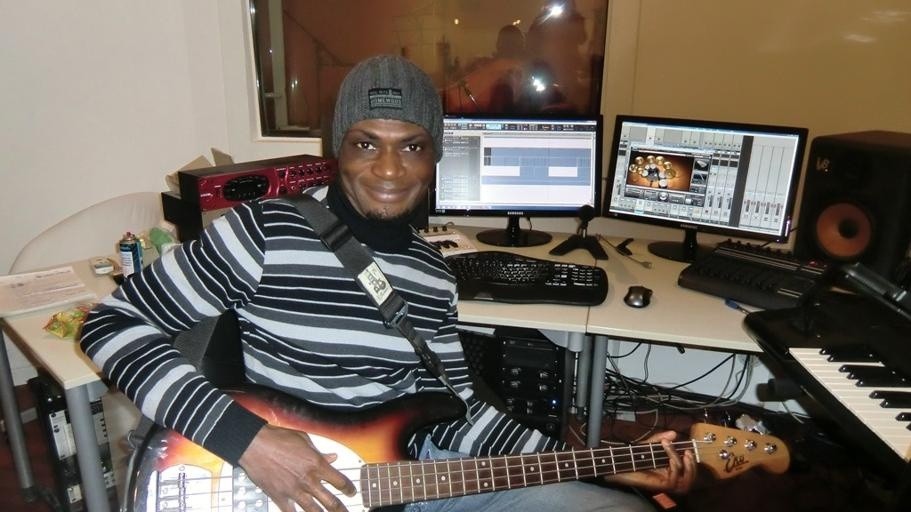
0 266 94 319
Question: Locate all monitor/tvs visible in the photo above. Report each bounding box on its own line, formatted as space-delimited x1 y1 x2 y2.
430 112 604 247
602 112 809 263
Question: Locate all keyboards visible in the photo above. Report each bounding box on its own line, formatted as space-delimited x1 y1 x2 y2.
443 250 609 306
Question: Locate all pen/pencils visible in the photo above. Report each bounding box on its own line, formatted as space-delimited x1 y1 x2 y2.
725 299 753 317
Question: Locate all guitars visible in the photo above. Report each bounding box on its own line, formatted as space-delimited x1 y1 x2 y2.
123 382 791 512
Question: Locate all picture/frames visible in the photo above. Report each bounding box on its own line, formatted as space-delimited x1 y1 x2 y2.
252 1 608 137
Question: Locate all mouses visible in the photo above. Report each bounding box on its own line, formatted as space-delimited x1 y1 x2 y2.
624 285 653 308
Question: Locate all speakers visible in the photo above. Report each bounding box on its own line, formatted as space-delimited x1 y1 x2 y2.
794 129 911 283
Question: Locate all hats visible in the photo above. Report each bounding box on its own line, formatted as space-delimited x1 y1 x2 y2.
331 54 444 162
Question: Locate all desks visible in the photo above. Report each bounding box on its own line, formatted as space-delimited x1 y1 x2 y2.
587 233 763 451
1 225 595 511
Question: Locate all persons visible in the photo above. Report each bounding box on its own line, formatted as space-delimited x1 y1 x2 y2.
77 53 697 511
453 0 588 115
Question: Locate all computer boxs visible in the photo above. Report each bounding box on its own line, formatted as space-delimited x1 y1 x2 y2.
491 326 576 442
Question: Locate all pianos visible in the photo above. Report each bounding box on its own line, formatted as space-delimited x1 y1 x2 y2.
744 306 911 475
